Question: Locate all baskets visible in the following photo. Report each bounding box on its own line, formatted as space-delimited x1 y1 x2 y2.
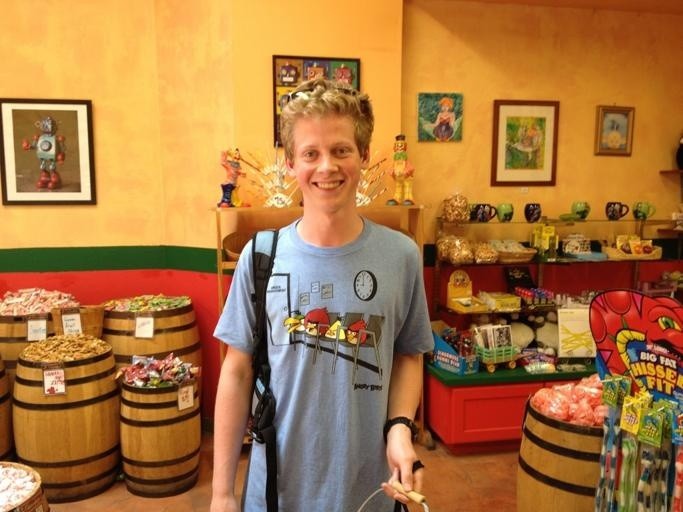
499 248 538 264
223 230 256 261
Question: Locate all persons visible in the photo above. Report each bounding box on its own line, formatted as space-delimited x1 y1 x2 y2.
209 79 435 512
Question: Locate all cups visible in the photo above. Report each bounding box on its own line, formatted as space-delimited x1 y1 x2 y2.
476 203 497 222
525 204 541 223
606 203 629 220
633 202 655 220
497 204 513 223
572 202 591 219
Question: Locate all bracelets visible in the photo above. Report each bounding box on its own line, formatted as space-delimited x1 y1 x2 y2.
383 416 420 443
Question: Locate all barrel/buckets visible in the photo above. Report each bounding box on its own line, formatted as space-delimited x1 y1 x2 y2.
516 395 603 512
0 305 103 394
101 303 202 367
12 355 120 503
0 355 13 462
0 462 51 512
120 376 201 498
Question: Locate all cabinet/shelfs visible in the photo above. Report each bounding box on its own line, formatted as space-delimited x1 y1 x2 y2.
436 218 683 316
215 203 425 443
426 370 597 455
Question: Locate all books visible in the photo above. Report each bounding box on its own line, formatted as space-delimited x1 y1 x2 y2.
472 323 512 348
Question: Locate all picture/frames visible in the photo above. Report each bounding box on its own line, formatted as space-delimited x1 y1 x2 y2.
272 55 360 147
594 106 635 156
490 100 559 187
0 99 95 204
